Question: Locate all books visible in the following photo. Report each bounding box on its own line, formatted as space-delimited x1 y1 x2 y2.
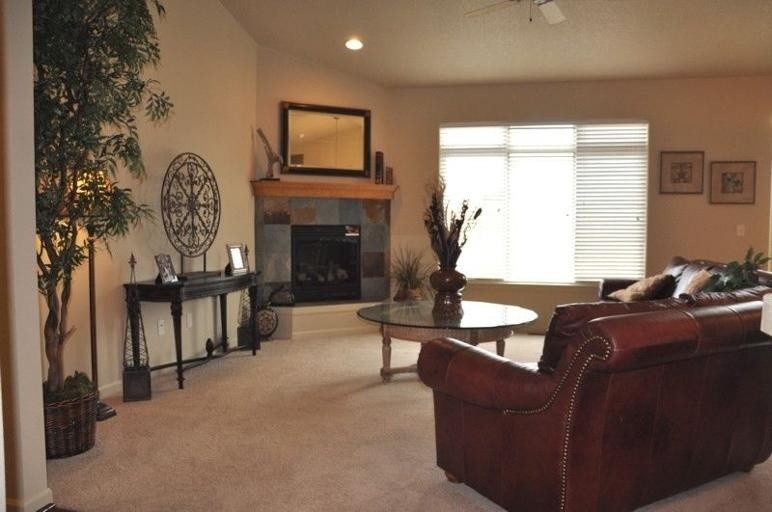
386 167 393 185
375 151 383 184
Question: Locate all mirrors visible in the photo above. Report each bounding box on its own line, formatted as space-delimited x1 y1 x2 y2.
281 101 370 177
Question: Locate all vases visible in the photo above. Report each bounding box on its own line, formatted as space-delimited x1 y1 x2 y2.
430 263 467 302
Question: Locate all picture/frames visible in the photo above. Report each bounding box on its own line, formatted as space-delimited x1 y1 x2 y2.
223 242 247 275
154 254 179 284
660 151 704 194
710 160 756 204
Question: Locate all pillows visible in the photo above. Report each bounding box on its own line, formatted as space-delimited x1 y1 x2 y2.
608 274 672 302
686 269 712 293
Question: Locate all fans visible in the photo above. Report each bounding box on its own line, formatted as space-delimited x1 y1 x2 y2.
462 0 568 26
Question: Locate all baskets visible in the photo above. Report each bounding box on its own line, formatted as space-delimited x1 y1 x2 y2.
43 383 102 460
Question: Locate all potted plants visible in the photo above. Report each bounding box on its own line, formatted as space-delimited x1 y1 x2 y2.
382 241 437 301
31 0 173 460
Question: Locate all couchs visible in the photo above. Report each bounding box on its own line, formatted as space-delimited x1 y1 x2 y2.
418 257 772 512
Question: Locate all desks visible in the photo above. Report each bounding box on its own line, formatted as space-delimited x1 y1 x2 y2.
123 270 261 389
